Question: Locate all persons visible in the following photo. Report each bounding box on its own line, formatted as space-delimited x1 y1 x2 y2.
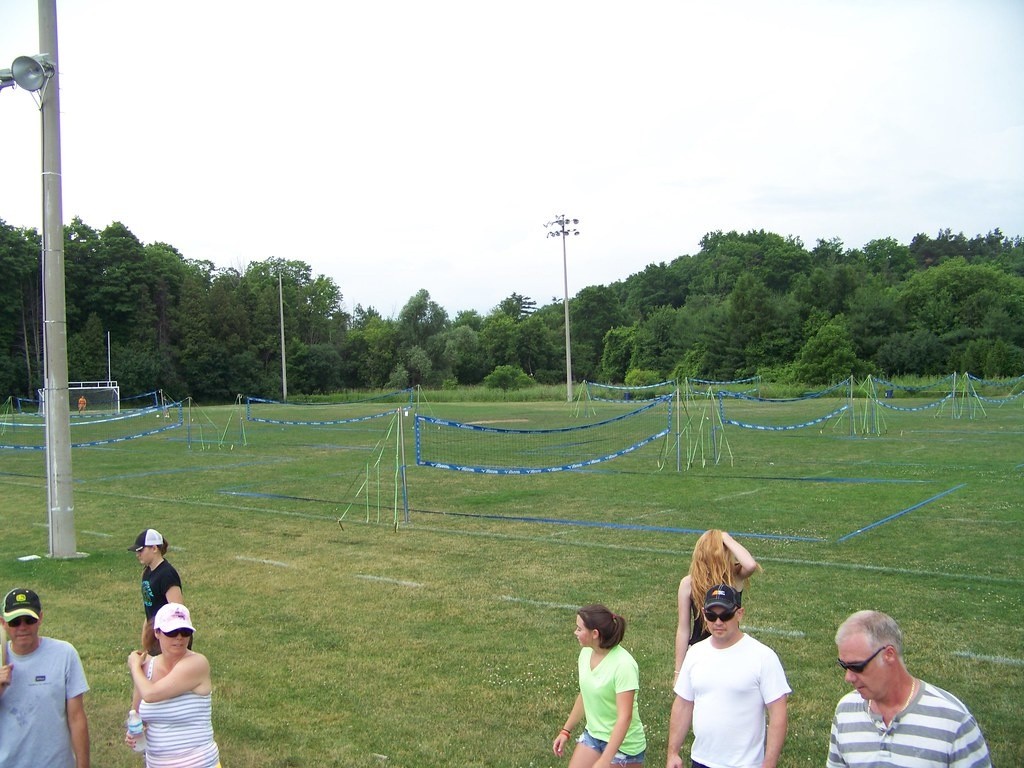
126 603 223 768
824 610 992 768
0 587 92 768
553 604 646 768
667 584 792 768
78 396 86 414
127 528 193 652
673 529 756 687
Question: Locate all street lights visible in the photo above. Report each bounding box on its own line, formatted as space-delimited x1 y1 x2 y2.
543 213 581 403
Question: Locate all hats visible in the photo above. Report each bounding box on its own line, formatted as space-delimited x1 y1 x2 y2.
154 603 196 632
128 528 163 551
703 585 742 609
3 588 41 622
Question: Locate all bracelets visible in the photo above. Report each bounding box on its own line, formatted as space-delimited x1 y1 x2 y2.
675 671 679 673
560 732 570 738
563 729 570 733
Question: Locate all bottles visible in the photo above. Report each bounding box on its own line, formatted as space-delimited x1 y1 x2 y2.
126 709 147 753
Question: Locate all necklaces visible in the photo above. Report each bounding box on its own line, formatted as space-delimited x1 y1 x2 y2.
868 677 916 726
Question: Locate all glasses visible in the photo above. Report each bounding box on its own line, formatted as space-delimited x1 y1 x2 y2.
838 645 893 674
9 616 38 627
162 628 193 637
703 607 739 622
